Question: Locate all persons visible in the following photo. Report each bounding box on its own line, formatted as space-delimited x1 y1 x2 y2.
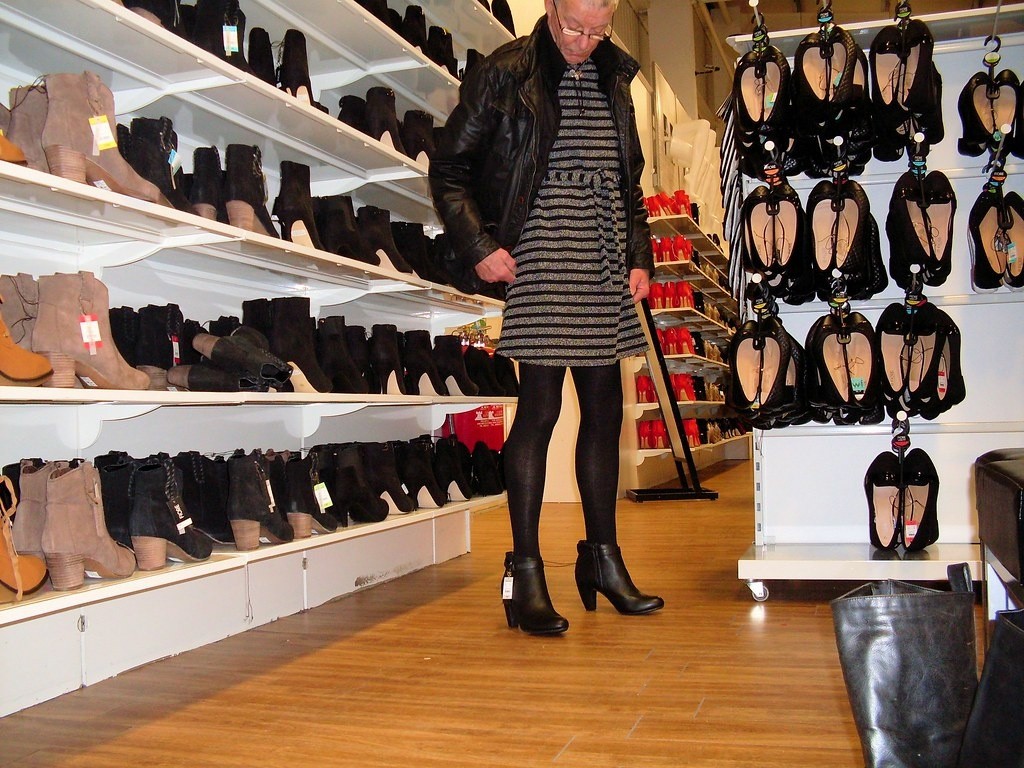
427 0 664 634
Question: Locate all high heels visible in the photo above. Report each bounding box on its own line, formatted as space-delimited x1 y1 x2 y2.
574 540 664 616
638 189 746 449
501 552 569 634
864 448 940 552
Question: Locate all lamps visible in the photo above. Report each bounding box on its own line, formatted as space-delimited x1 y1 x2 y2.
695 65 720 76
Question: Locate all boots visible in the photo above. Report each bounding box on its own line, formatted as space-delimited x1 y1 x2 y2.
0 0 516 596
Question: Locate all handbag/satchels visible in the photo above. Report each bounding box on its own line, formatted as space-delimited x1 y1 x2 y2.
833 562 978 768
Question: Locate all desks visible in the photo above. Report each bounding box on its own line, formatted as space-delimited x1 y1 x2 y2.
975 448 1024 682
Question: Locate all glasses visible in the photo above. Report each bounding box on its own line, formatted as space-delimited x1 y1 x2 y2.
553 0 614 41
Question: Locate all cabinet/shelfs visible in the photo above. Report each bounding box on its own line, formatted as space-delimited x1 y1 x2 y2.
0 0 519 720
622 216 731 491
731 0 1024 602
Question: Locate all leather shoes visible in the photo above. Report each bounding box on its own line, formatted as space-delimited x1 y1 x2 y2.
732 20 1024 430
